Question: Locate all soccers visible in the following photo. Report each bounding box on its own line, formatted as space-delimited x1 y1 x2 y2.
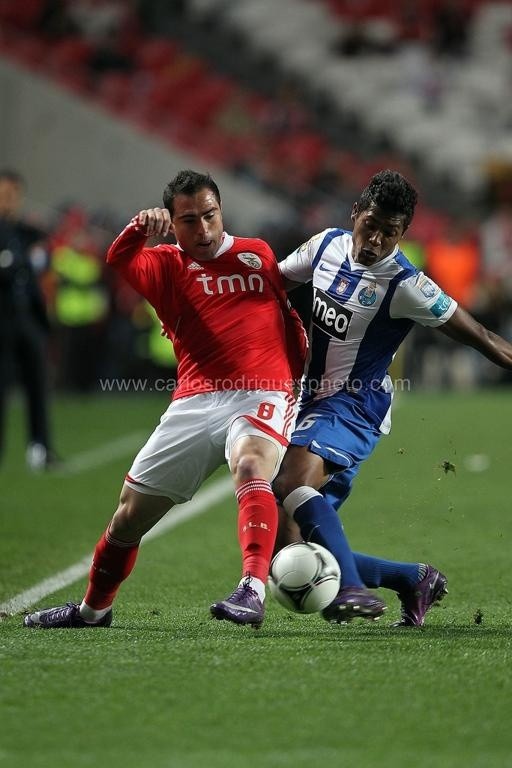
267 540 341 614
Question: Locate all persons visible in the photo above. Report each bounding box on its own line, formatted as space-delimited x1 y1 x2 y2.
275 166 511 633
20 165 312 633
0 170 65 471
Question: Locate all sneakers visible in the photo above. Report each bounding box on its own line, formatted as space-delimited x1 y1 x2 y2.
322 585 387 623
208 570 266 630
22 601 114 629
388 563 449 630
